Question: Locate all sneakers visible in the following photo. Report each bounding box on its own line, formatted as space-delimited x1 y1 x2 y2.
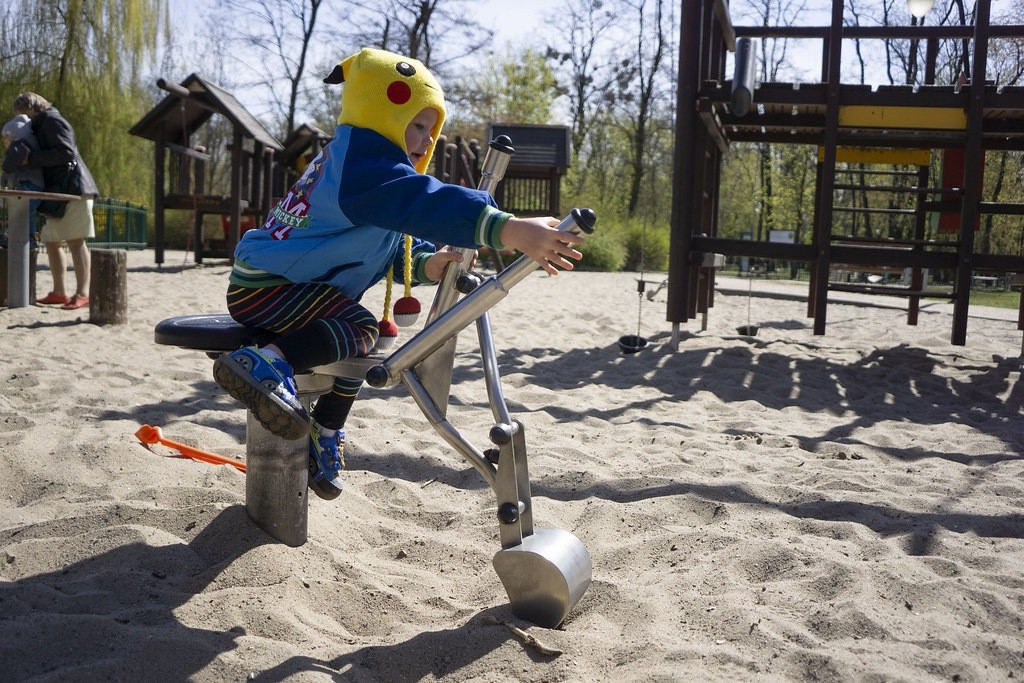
212 347 310 440
308 416 346 500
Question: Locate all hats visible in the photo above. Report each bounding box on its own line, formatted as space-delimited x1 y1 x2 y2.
322 48 447 351
2 114 31 137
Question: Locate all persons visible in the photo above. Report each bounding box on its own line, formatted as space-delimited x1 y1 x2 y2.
3 92 99 310
212 48 585 504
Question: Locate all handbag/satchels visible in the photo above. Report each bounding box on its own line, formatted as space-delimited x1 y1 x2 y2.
37 163 74 217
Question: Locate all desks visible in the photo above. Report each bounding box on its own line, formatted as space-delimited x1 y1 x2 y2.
0 189 82 310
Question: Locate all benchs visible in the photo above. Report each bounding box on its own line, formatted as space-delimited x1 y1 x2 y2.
971 276 997 288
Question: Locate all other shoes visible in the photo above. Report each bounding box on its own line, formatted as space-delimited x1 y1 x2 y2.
60 296 89 310
35 292 68 308
